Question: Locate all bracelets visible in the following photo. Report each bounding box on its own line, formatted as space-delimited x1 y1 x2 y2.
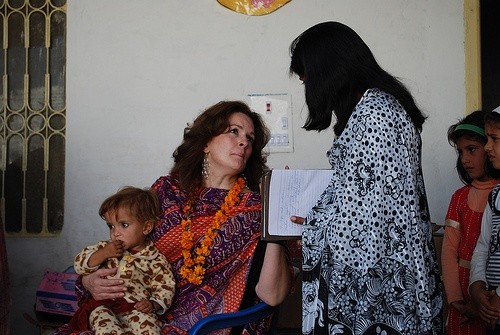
151 301 156 314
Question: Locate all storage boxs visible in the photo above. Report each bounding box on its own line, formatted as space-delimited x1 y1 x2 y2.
34 271 83 317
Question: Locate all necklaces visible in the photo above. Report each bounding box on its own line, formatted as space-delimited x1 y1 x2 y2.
180 178 246 285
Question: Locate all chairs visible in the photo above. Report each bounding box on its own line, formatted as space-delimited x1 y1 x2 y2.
190 292 281 335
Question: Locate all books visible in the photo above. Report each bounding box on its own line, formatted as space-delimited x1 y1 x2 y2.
261 169 338 241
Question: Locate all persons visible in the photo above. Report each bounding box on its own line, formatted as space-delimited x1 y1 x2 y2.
74 185 175 335
51 101 296 335
467 106 500 335
441 111 500 335
290 21 445 335
